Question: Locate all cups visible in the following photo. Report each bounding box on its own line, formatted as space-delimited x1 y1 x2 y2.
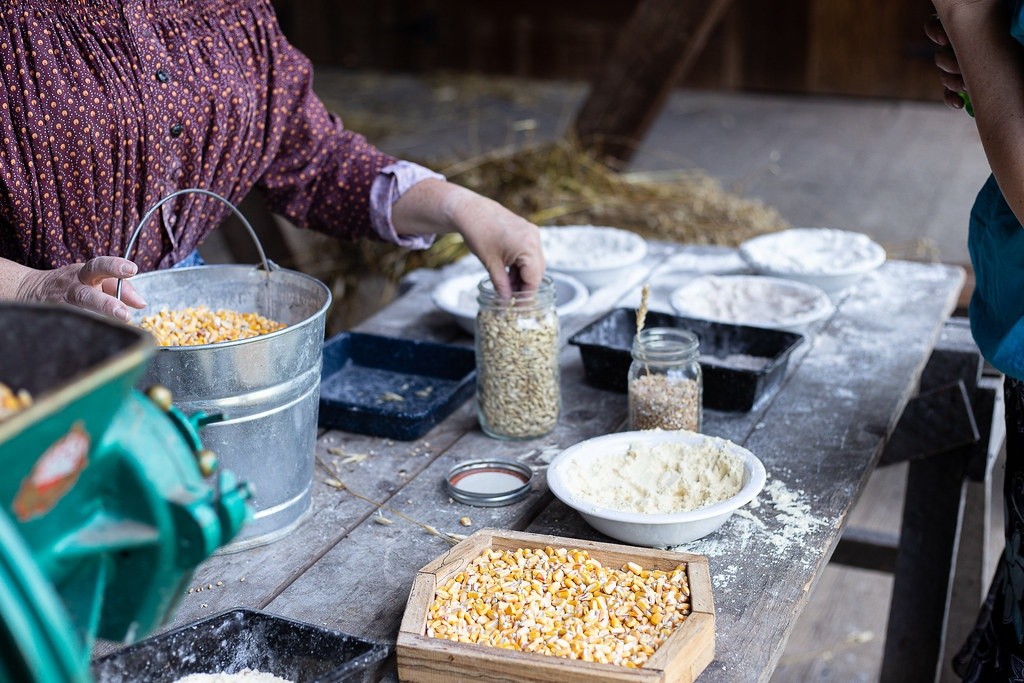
628 326 703 432
475 275 561 441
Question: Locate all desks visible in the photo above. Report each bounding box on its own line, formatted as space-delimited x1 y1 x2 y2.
92 217 971 683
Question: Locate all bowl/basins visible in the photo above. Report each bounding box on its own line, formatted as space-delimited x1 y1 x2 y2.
532 224 647 288
669 273 831 334
546 424 767 548
430 270 589 326
739 227 888 291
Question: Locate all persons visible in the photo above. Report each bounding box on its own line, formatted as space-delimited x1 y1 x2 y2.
920 0 1023 683
0 0 549 335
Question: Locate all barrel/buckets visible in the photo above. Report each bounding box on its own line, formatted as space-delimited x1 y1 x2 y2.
94 188 332 558
